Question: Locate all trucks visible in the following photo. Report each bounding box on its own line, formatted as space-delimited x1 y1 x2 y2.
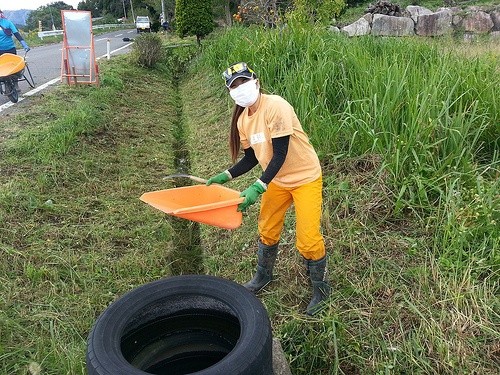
136 15 150 33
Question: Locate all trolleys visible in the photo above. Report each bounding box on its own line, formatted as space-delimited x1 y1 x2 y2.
0 48 37 103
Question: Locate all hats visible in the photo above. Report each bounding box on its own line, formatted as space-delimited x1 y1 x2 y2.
224 66 257 87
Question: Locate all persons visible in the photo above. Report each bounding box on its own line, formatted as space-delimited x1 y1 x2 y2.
206 61 332 317
0 8 30 92
163 19 172 31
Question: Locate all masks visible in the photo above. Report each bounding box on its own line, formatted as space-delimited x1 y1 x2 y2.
229 78 259 107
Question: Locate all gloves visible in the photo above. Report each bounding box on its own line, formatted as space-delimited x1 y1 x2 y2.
206 171 230 187
237 182 267 211
19 40 30 52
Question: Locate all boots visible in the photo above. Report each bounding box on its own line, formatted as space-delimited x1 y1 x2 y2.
303 256 330 315
241 241 279 293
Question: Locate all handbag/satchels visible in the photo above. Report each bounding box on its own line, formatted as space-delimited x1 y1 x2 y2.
0 24 12 36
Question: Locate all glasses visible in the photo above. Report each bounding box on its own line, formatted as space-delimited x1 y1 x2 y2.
222 62 247 80
